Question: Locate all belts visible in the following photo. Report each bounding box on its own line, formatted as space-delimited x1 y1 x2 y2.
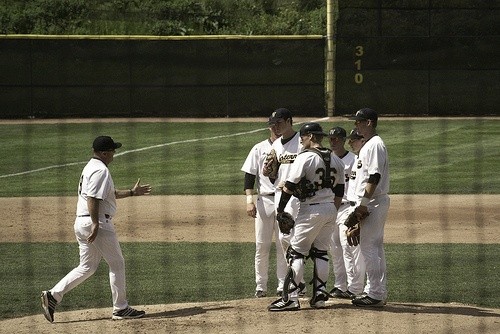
267 193 276 196
79 214 109 219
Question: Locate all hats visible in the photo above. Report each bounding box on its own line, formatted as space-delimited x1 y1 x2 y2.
327 127 346 138
93 136 122 151
267 115 275 123
345 129 364 139
348 108 377 121
270 108 292 120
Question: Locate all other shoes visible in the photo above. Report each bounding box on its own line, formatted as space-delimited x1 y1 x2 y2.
255 291 266 298
298 292 305 297
277 291 283 297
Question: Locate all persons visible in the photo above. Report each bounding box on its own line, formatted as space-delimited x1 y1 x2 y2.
262 108 308 297
41 136 170 323
240 116 288 297
267 122 345 309
352 107 390 306
327 126 366 300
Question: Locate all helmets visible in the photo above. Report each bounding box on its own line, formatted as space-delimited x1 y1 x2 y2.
300 123 328 136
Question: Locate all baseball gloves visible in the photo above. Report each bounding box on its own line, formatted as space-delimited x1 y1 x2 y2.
275 212 295 236
293 177 309 200
345 223 361 247
262 149 279 180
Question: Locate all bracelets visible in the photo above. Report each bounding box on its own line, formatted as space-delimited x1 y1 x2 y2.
246 195 252 203
360 197 369 207
130 189 133 196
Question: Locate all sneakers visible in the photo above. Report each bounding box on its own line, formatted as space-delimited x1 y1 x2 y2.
310 294 325 308
41 291 57 323
112 307 145 320
333 290 355 300
330 290 341 297
268 299 299 311
352 296 384 307
